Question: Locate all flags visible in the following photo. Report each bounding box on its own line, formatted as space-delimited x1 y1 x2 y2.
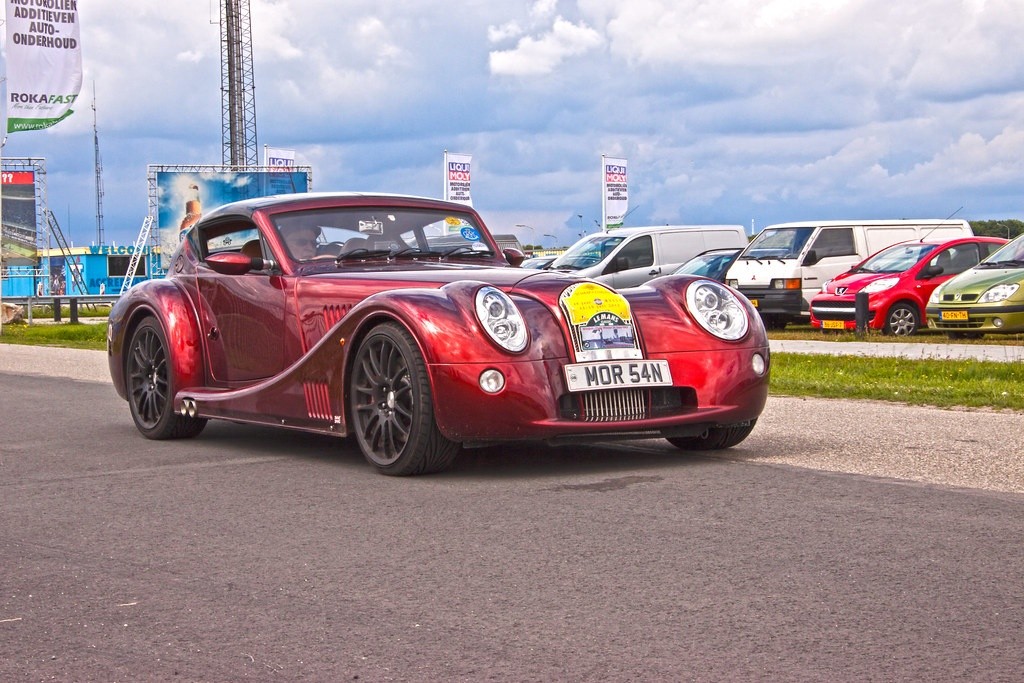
605 158 627 228
5 0 82 133
446 153 474 235
267 147 295 195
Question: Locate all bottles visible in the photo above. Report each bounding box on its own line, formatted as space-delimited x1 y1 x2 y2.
179 184 202 242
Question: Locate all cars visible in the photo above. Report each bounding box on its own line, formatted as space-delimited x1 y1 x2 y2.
668 245 791 285
926 232 1023 339
809 237 1023 338
520 254 604 269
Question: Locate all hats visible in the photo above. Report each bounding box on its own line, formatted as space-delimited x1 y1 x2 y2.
279 220 321 238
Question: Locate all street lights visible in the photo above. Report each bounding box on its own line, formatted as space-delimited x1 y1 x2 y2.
517 224 535 250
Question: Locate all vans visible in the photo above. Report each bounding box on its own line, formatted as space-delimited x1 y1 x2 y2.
544 224 750 292
724 218 975 331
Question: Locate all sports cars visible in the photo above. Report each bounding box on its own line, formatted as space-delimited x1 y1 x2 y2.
107 192 771 477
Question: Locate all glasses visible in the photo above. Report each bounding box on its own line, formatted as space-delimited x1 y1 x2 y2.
285 238 320 248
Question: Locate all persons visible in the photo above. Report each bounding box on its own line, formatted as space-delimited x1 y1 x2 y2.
52 275 60 295
279 221 322 259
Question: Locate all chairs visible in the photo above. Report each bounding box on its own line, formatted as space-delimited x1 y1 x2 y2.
935 250 951 270
240 240 266 260
340 236 366 255
952 248 977 269
